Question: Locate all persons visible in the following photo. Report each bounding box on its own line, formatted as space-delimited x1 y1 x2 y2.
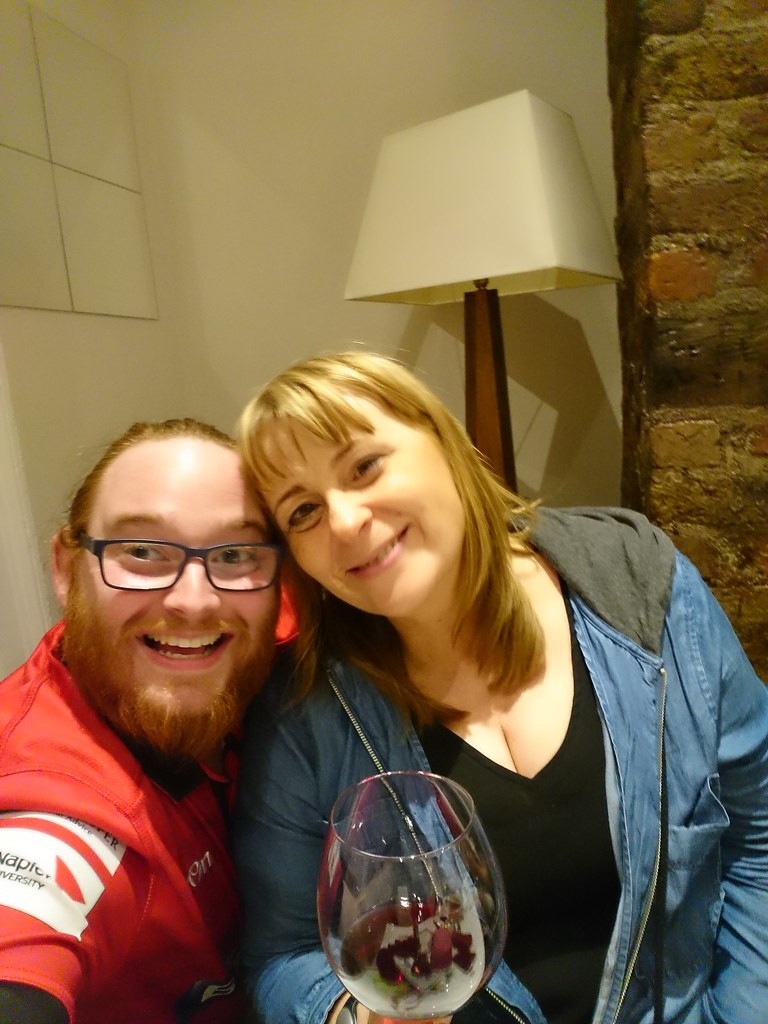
228 348 768 1024
0 418 288 1024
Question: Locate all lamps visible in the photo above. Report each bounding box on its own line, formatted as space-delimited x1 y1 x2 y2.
344 89 627 501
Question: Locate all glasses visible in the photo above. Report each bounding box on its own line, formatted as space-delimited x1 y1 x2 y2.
79 530 284 593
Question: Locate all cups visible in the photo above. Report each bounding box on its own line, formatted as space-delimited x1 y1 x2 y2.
315 770 505 1024
